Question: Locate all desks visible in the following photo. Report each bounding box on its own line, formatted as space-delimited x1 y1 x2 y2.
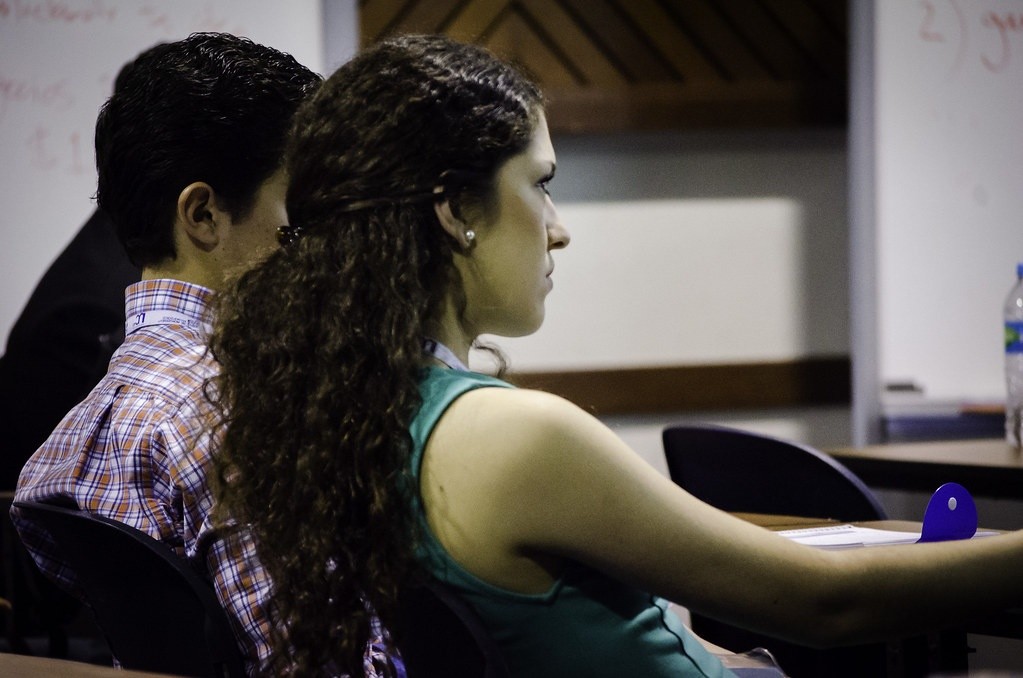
814 439 1023 502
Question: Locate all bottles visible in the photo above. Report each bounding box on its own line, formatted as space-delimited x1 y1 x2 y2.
1004 263 1023 449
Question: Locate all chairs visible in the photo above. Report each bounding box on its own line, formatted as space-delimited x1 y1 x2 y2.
652 421 970 674
10 500 254 677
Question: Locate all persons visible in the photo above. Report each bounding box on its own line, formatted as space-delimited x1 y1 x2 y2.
202 33 1023 678
0 34 406 678
